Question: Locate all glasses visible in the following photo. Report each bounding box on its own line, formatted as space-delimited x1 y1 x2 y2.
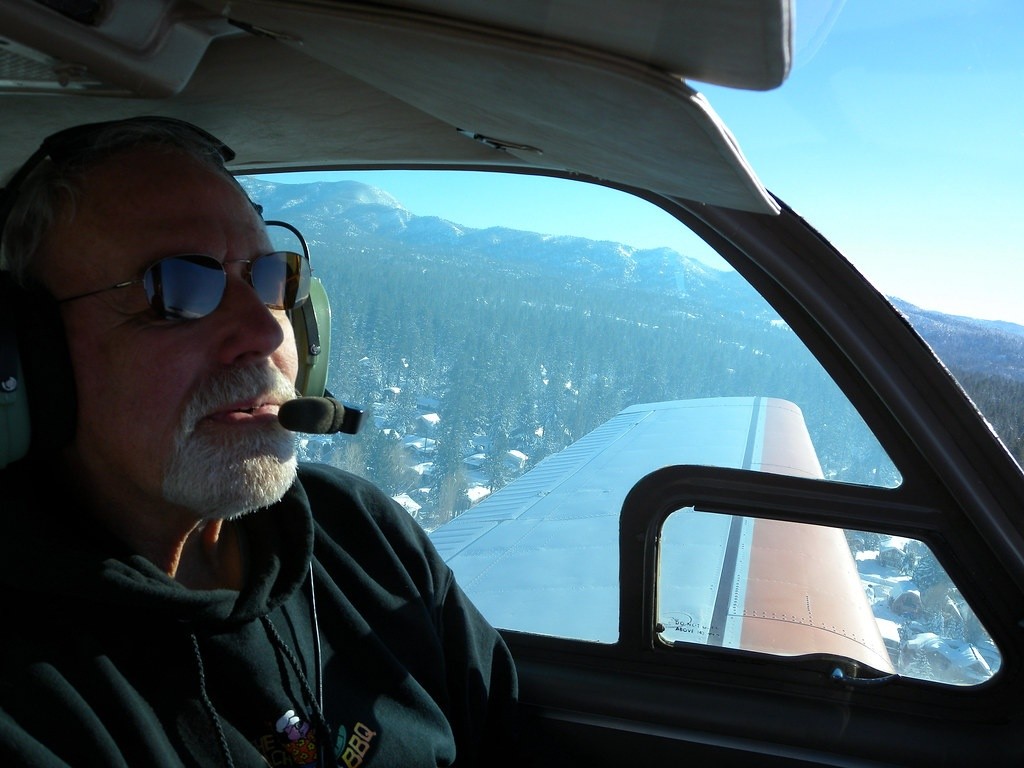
58 251 311 321
0 115 236 212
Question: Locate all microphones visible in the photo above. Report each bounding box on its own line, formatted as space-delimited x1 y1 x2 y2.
279 388 362 435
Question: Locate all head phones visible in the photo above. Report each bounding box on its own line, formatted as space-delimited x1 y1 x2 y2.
0 116 330 468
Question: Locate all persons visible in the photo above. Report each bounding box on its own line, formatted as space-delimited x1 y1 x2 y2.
0 115 525 768
